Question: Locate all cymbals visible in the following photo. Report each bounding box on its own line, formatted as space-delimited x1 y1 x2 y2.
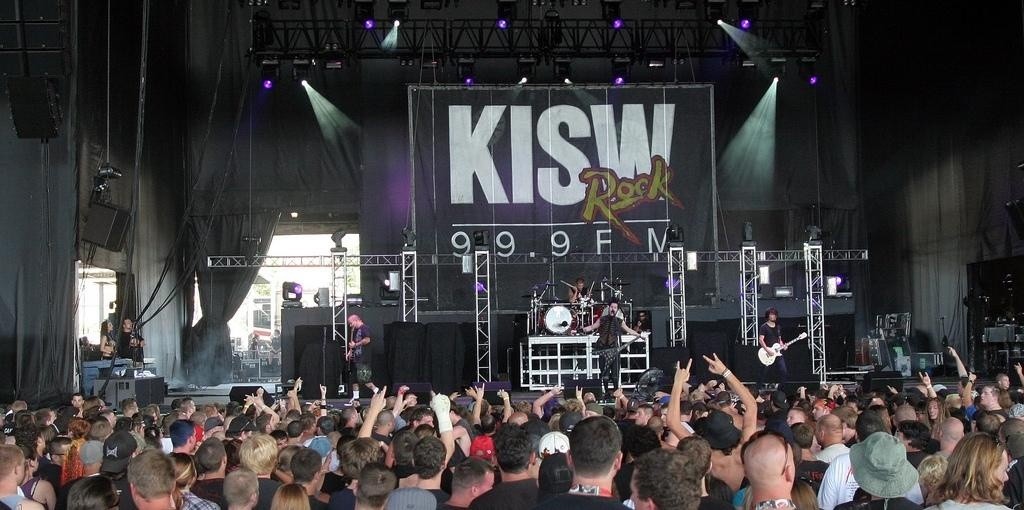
527 287 542 292
520 293 542 298
610 281 630 286
594 288 609 291
597 280 613 283
537 283 557 285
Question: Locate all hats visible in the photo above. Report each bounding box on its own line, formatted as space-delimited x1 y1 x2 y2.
691 410 742 450
538 410 583 494
204 417 224 432
230 416 256 431
850 431 919 499
772 390 790 409
101 430 136 473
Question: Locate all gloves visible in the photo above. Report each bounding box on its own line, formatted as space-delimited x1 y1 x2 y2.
430 393 453 434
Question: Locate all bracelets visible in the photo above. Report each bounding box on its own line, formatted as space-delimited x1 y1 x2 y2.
354 342 356 347
576 328 583 333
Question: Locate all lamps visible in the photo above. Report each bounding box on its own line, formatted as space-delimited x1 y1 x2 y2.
326 219 352 252
376 279 390 295
835 274 850 292
399 224 417 250
771 286 794 300
665 224 684 247
283 282 303 308
475 229 491 249
651 278 680 302
803 222 821 244
740 223 756 245
469 281 495 305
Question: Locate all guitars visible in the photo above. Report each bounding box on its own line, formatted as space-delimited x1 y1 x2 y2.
758 331 808 366
590 331 651 379
347 320 356 373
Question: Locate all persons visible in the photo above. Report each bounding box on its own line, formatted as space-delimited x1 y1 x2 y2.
119 318 145 362
343 314 381 407
633 311 651 332
569 278 591 303
268 329 281 366
571 302 646 399
0 351 1023 510
759 307 787 386
100 321 116 360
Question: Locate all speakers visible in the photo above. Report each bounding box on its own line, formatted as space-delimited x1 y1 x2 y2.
82 201 132 252
564 379 602 400
472 381 511 405
393 383 432 404
4 76 64 139
863 371 903 393
229 386 274 407
93 376 164 411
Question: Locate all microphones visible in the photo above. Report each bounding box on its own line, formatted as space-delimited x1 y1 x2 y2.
323 327 327 336
612 309 616 315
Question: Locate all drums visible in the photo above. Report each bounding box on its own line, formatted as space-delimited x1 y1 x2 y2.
588 304 627 335
543 306 579 335
575 297 592 312
